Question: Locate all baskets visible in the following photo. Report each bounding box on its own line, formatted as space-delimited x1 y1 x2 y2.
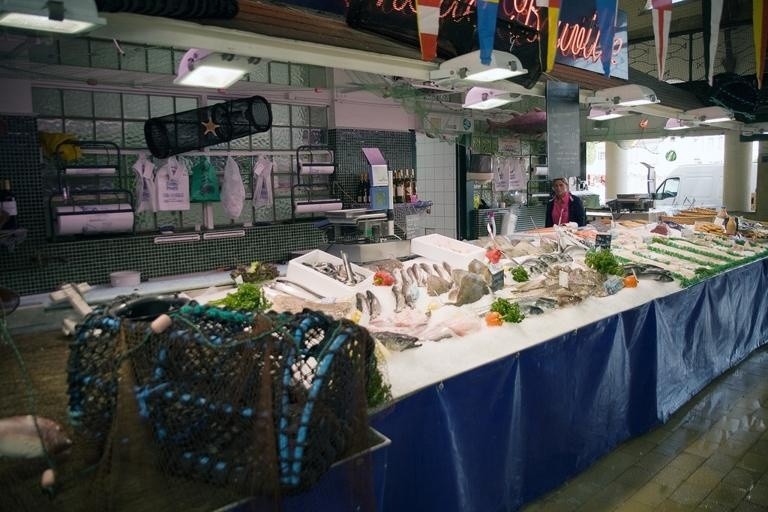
680 208 718 215
661 215 695 225
673 213 716 222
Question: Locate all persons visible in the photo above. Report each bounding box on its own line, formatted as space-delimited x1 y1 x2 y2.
544 177 586 229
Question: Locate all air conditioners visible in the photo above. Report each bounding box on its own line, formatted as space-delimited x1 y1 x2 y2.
414 109 476 136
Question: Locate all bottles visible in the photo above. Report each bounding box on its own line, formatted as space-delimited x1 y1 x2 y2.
362 174 369 203
357 174 363 203
393 168 417 204
726 217 736 235
716 206 729 230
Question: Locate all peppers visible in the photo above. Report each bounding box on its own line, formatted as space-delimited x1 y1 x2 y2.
371 270 395 287
485 311 505 328
486 249 502 264
625 275 639 288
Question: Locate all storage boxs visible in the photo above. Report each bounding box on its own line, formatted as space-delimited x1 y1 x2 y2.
412 233 486 268
286 248 375 305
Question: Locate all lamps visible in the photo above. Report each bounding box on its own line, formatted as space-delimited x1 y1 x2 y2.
461 85 521 110
585 107 629 123
174 54 266 92
684 106 737 128
662 118 691 132
429 50 529 83
0 0 107 39
587 84 661 109
